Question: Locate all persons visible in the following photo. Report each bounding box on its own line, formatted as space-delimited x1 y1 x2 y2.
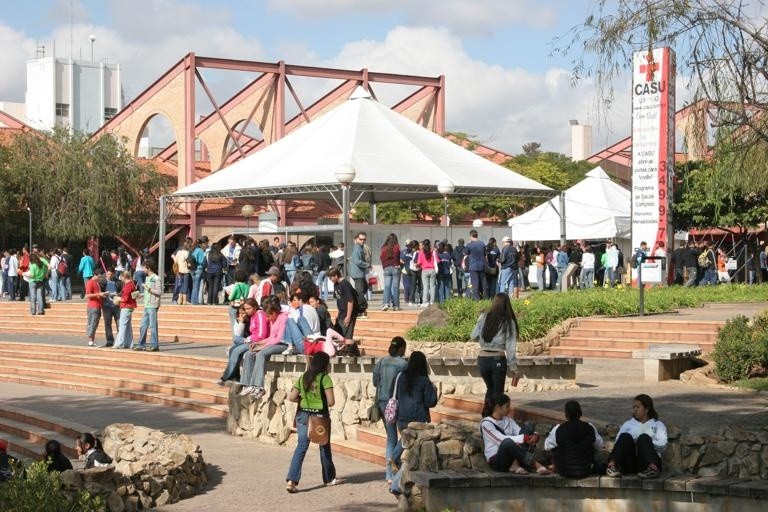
673 240 731 287
346 230 373 320
379 234 401 311
655 241 667 267
606 394 669 477
519 238 622 290
544 400 603 478
0 438 26 480
749 254 756 284
403 231 521 306
95 246 155 294
44 440 73 485
78 249 95 298
636 241 647 287
286 351 347 492
217 266 361 401
18 254 47 315
373 336 409 484
470 293 520 417
388 351 438 500
1 244 71 303
760 247 768 282
76 433 113 469
84 259 162 352
171 235 348 306
480 394 548 475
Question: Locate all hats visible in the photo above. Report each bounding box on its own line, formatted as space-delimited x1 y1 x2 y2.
502 237 511 241
264 266 281 276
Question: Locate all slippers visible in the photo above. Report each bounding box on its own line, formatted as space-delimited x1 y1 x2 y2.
286 486 298 493
508 467 528 474
324 478 346 487
536 465 549 475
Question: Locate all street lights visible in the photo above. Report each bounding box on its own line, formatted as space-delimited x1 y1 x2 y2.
26 207 33 253
240 204 257 236
333 162 356 283
471 217 486 230
436 177 454 245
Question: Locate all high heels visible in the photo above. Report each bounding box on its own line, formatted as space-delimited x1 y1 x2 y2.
385 457 399 474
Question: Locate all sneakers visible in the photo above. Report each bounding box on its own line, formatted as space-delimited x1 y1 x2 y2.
606 465 621 477
88 339 158 352
282 348 296 355
637 466 659 479
216 380 265 400
382 302 434 311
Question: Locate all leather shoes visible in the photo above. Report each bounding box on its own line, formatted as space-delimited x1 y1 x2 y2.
389 489 399 499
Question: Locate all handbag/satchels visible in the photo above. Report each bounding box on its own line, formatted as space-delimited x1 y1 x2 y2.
410 261 419 271
340 280 367 313
629 254 638 268
308 416 331 446
365 267 380 291
186 256 197 269
173 262 179 274
384 398 398 424
366 405 381 422
434 257 438 274
484 264 497 275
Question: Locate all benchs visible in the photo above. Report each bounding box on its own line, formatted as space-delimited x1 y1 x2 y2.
268 355 584 389
408 467 767 512
633 345 701 381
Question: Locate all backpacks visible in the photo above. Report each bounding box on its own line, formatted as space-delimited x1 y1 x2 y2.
53 255 70 277
698 249 711 268
